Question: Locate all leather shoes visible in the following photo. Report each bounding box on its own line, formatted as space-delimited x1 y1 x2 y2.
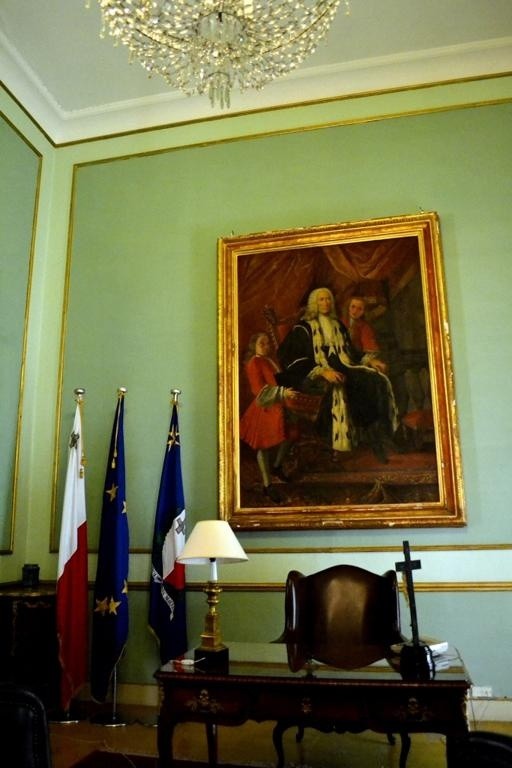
269 464 289 483
263 483 283 503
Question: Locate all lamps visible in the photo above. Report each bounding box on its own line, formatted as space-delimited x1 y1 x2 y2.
82 0 350 110
175 520 249 670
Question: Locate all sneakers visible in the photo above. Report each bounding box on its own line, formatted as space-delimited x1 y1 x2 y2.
372 432 407 465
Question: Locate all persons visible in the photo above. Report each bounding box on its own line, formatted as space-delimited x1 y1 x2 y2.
277 283 388 449
343 297 420 418
238 331 292 487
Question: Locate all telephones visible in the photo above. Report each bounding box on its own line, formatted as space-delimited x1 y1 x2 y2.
391 637 449 658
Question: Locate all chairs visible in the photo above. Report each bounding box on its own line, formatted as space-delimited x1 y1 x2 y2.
1 686 51 768
270 564 411 768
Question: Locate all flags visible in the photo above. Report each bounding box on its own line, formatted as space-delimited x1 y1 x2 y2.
53 406 88 716
92 393 131 707
145 403 190 665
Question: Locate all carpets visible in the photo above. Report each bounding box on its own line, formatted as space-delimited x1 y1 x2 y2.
67 750 255 768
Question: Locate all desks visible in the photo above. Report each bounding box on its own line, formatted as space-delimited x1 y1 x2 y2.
152 640 472 768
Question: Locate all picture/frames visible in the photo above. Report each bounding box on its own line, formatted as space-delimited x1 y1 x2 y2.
217 210 468 532
